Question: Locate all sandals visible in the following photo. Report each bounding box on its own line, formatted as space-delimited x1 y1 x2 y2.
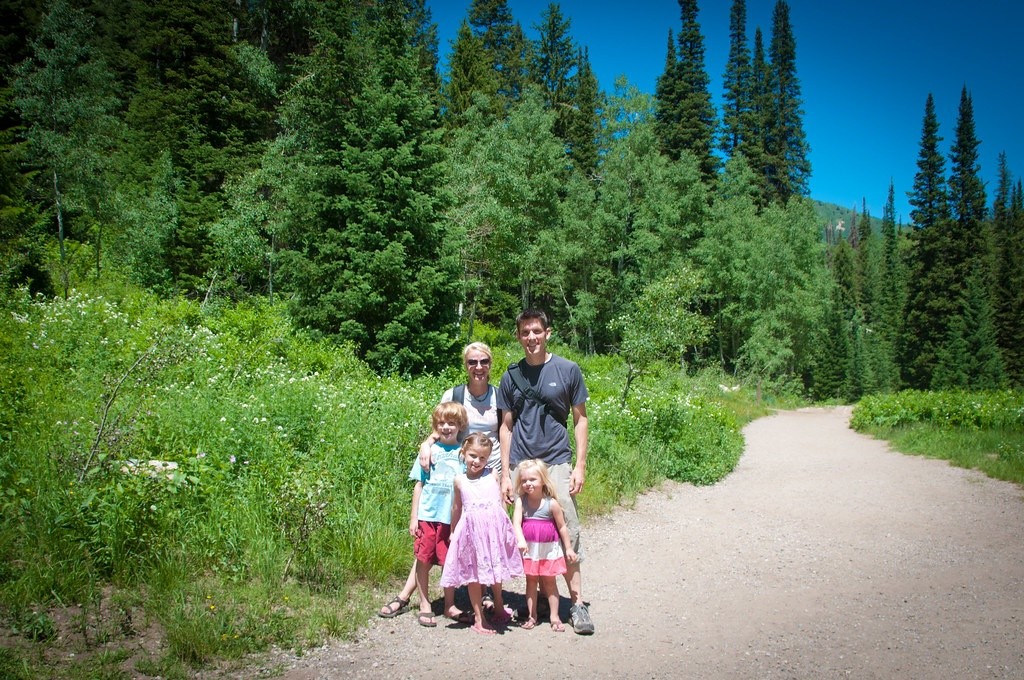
480 593 493 610
378 596 410 618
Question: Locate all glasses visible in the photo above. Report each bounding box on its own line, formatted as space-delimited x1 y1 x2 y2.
467 359 490 365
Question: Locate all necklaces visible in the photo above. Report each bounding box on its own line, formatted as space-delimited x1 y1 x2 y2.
467 383 490 403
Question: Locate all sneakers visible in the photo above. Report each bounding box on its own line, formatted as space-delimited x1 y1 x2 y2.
568 600 596 634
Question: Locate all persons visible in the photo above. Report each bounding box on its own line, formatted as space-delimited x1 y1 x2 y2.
378 341 509 618
409 401 473 627
511 458 578 632
439 433 523 634
496 308 595 636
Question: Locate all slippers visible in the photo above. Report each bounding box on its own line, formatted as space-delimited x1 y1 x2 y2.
452 611 472 623
491 608 513 624
471 625 497 635
550 621 565 632
521 620 535 629
418 612 437 627
